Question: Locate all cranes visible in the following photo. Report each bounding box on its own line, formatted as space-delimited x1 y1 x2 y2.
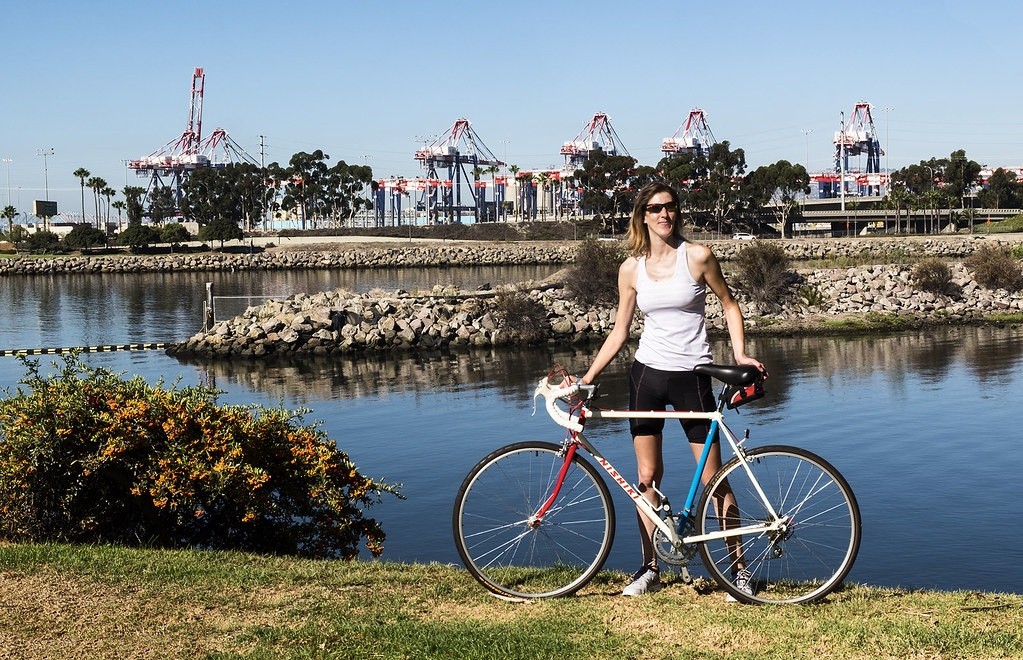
661 109 720 180
559 113 637 203
832 102 885 195
412 118 508 211
128 67 238 219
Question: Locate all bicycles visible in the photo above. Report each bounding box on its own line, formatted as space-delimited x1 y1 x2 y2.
453 362 863 606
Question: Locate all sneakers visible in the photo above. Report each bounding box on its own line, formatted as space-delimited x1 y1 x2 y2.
725 567 754 601
622 563 661 596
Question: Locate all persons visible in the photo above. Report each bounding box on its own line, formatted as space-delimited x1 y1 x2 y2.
560 181 768 603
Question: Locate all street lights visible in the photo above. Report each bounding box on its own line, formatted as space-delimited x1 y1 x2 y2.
14 187 23 225
849 193 861 237
414 134 437 224
882 107 896 196
924 165 934 195
2 158 14 206
359 155 373 227
36 147 55 231
801 129 813 174
499 140 512 221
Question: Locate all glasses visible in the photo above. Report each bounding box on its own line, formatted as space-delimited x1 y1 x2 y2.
645 201 677 213
